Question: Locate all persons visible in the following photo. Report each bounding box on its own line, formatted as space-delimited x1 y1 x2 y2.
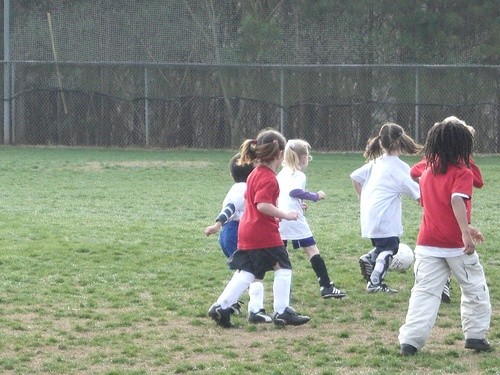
350 123 421 293
207 130 311 327
276 139 346 298
398 116 491 356
205 152 273 323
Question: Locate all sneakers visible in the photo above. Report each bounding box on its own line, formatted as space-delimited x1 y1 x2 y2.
247 308 271 322
272 307 310 324
401 344 417 354
442 285 450 302
465 338 494 352
227 302 245 314
322 286 345 297
360 254 376 281
209 304 235 327
365 279 397 293
391 261 411 271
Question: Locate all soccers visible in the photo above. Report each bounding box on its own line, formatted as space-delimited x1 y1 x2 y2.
389 243 414 272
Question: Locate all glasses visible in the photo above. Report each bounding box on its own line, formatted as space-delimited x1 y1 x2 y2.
305 155 313 162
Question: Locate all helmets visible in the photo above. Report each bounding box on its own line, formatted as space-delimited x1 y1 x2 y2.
229 153 254 176
